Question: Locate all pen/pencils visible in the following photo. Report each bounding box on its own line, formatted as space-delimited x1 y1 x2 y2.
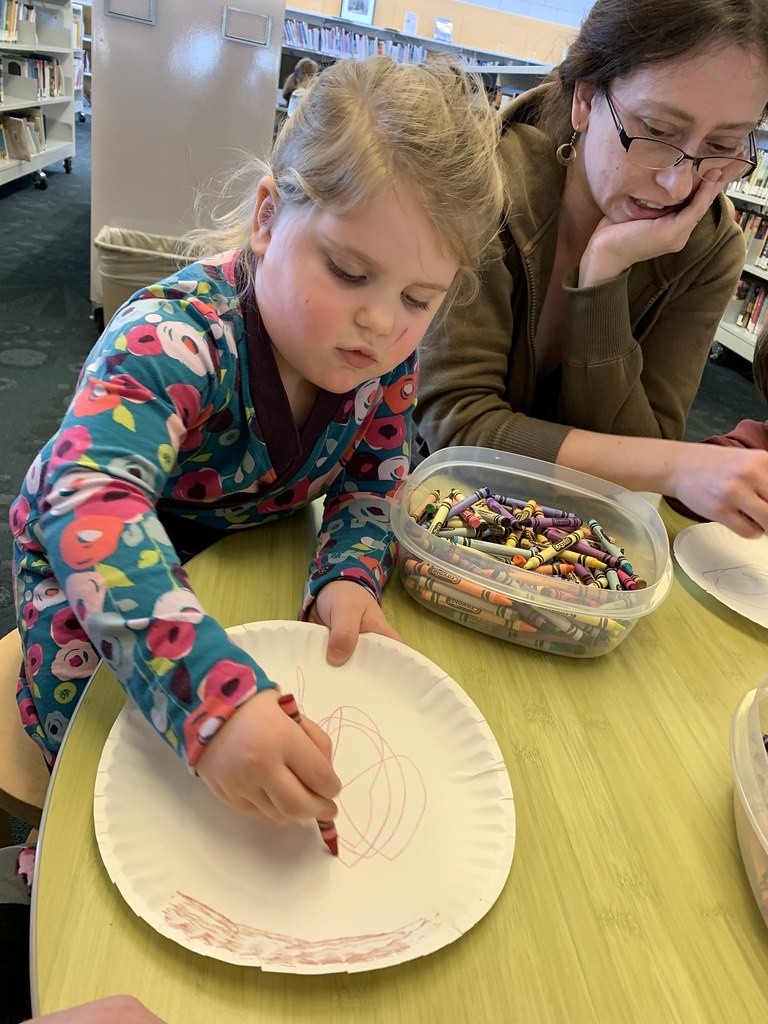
282 19 500 67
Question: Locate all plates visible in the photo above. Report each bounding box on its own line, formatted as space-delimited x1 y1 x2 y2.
671 516 768 631
94 618 517 977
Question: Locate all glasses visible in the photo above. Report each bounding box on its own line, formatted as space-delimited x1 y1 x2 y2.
601 80 758 182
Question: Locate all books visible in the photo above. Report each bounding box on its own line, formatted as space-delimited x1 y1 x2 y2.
272 17 527 110
709 127 768 333
0 0 91 167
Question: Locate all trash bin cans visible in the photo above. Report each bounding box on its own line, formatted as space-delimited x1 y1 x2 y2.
93 225 204 329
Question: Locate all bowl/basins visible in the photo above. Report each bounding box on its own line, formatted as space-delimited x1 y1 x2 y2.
729 670 767 932
389 443 674 660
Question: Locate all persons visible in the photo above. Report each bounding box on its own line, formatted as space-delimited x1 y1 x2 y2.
662 323 768 526
281 58 322 121
5 55 507 890
411 0 768 543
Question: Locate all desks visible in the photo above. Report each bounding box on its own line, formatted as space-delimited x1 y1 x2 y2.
23 474 768 1024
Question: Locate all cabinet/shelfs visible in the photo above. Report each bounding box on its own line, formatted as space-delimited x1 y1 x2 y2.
0 0 76 195
271 7 768 364
